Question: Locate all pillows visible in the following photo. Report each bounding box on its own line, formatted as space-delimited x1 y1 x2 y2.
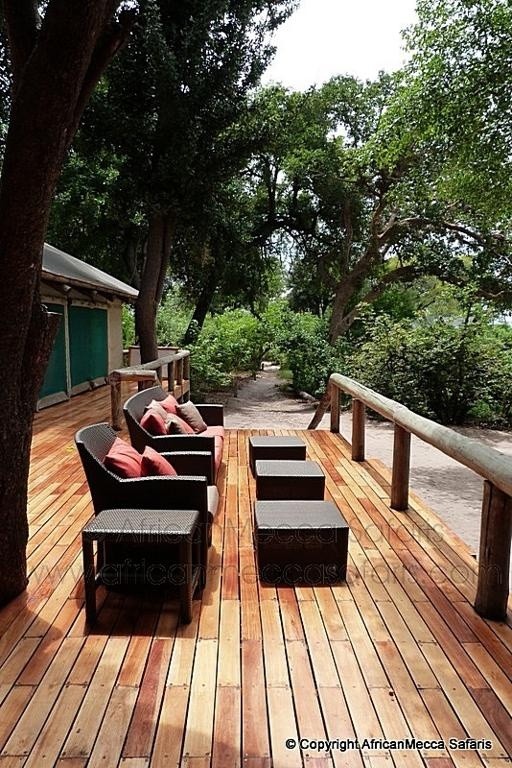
101 437 143 478
141 445 178 477
139 394 207 436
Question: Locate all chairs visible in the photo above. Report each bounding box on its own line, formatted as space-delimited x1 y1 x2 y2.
73 421 220 547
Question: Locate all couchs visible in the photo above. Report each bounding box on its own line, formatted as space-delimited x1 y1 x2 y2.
123 385 224 485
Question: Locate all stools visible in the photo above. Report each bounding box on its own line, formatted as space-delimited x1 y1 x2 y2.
255 459 325 500
248 436 306 479
253 501 349 588
